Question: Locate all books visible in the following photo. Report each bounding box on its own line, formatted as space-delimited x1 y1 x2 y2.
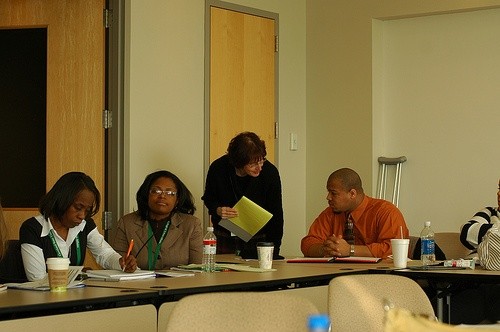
287 256 382 264
85 268 156 282
217 196 273 243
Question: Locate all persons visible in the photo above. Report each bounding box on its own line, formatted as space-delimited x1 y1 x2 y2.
459 178 500 266
201 130 286 260
112 171 203 270
18 172 138 282
300 168 412 261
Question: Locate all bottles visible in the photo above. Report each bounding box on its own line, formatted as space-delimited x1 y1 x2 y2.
420 222 436 265
201 227 217 273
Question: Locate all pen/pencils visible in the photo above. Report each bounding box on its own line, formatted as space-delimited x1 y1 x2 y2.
122 239 134 272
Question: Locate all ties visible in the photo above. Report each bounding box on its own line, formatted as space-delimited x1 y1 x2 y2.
343 213 355 244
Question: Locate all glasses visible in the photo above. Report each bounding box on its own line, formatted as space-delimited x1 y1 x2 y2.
150 186 177 198
247 158 266 169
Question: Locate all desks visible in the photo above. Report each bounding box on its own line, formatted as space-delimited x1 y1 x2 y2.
0 259 500 332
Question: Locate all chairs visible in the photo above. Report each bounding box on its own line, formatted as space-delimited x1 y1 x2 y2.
166 292 317 332
434 232 472 259
327 274 436 332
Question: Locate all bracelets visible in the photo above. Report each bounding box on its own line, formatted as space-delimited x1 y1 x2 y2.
350 245 355 257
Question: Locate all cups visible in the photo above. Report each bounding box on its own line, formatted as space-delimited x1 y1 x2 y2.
256 242 274 269
390 238 410 268
46 257 70 293
